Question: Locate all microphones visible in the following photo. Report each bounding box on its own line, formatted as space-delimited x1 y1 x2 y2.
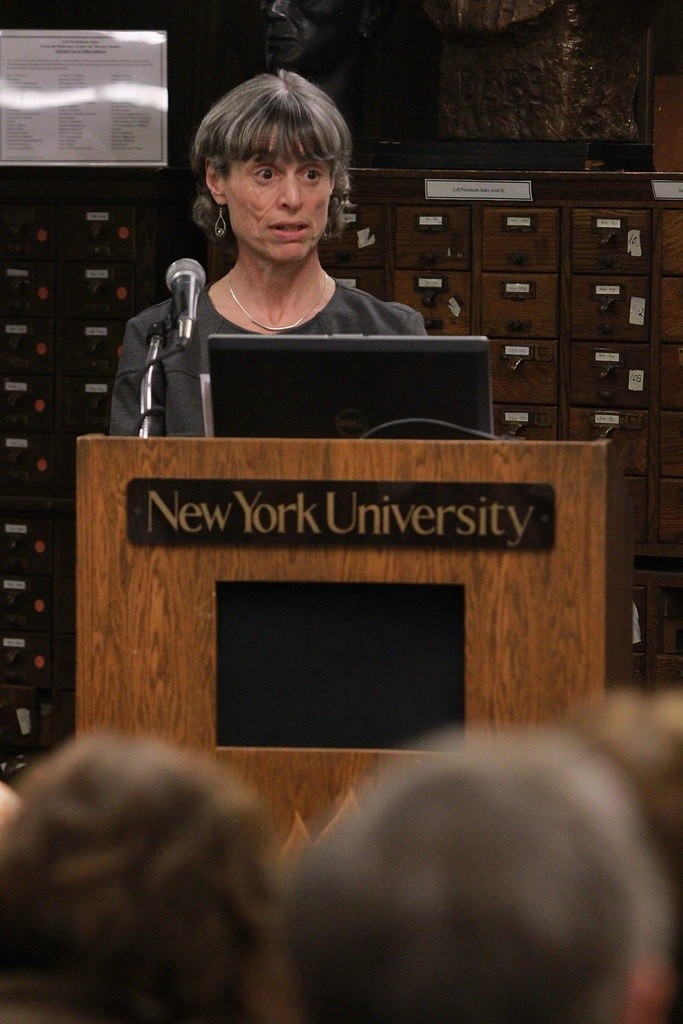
166 258 206 351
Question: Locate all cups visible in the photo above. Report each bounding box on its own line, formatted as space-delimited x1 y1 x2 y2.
199 373 215 437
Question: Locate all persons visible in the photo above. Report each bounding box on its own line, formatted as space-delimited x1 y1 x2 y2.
582 690 683 1024
293 736 669 1024
0 733 277 1024
108 69 427 437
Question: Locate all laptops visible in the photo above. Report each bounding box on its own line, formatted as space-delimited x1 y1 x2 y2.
205 333 494 443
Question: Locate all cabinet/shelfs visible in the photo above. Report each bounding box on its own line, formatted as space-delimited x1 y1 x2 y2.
0 167 683 789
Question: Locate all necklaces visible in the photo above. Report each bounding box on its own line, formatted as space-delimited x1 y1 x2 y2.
227 270 327 331
